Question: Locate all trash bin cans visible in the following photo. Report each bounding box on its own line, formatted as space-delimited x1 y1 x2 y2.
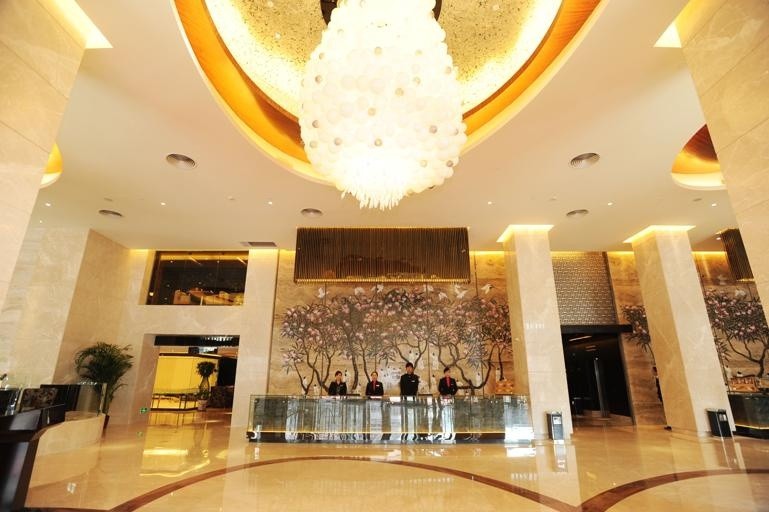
546 411 563 440
707 408 732 437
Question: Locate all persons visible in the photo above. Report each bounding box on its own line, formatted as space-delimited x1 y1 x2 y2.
652 367 663 407
366 372 384 396
439 368 458 396
400 362 418 396
328 371 347 396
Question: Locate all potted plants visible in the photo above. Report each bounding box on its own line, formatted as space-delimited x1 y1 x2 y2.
195 361 215 412
73 341 135 431
